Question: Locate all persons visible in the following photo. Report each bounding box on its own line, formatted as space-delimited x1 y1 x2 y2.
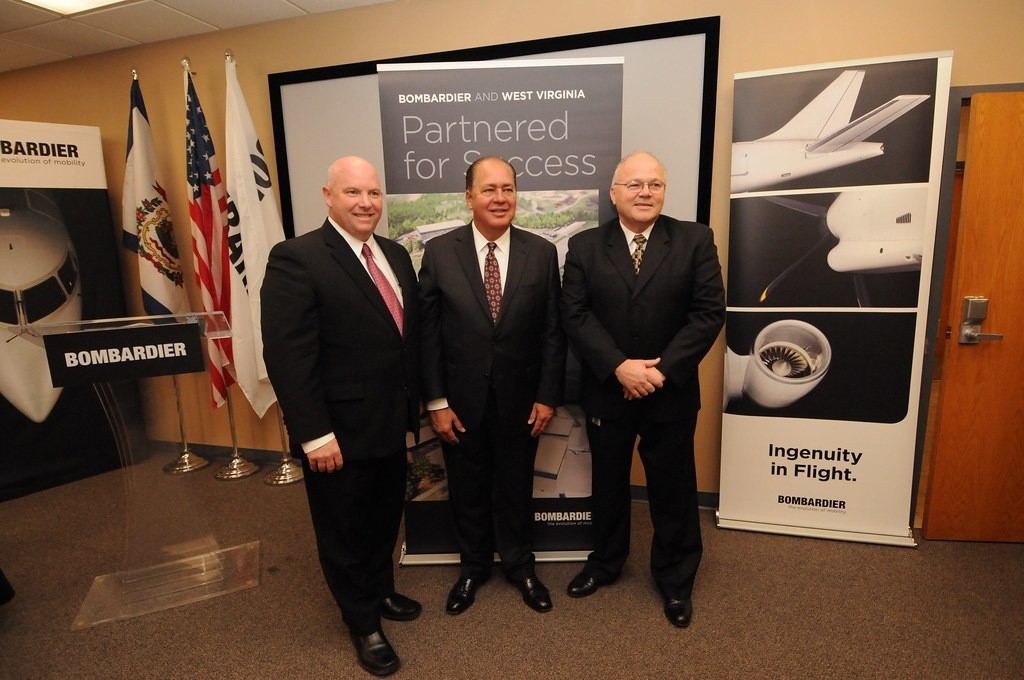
260 155 422 677
418 156 566 616
559 150 726 626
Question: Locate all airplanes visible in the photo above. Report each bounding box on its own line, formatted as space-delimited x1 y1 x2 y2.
729 70 932 195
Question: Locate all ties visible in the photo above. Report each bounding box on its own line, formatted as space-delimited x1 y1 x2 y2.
362 243 402 336
630 234 647 276
483 241 503 324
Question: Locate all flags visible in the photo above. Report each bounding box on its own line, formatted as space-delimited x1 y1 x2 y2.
122 75 193 325
184 69 240 409
224 60 285 420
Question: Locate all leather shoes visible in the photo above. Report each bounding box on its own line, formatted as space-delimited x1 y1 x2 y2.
568 570 600 597
349 625 401 677
664 597 693 628
379 591 421 621
513 573 553 613
446 574 480 615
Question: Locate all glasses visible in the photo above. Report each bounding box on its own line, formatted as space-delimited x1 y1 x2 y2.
613 179 667 193
